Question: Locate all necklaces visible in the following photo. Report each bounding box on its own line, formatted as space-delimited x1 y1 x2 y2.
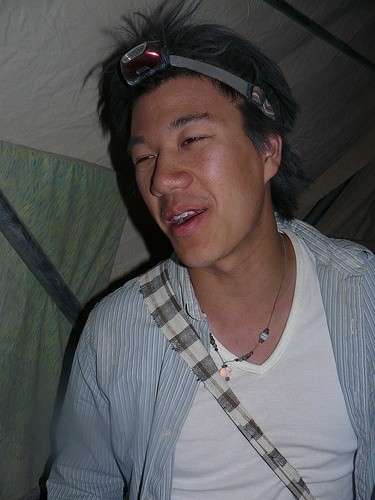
200 233 288 380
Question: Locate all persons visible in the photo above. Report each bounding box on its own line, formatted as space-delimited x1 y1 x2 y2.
46 0 375 500
